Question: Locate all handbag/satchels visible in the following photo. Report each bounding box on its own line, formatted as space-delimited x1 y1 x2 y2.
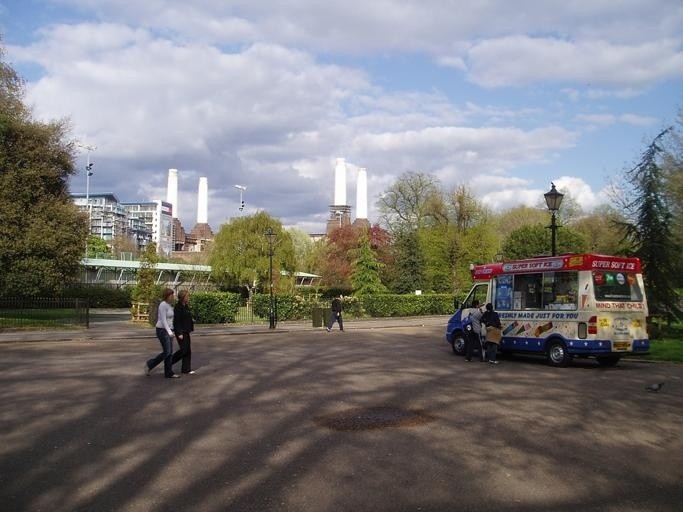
461 314 473 335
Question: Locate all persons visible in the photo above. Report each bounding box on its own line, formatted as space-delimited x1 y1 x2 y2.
326 293 345 333
480 303 503 364
171 290 196 375
144 288 182 377
464 299 487 363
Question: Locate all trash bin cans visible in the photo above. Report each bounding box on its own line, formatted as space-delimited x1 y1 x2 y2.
313 308 333 327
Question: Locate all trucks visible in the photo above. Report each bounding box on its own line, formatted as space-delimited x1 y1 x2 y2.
445 254 650 368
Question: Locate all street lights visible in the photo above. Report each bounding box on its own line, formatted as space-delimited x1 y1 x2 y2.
543 184 564 256
265 226 278 329
233 184 247 219
75 142 98 234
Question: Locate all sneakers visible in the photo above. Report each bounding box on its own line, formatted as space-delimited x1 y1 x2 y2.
326 327 345 332
144 363 197 378
463 357 499 365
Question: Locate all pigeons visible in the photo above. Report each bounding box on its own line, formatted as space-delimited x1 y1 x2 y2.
644 382 666 393
